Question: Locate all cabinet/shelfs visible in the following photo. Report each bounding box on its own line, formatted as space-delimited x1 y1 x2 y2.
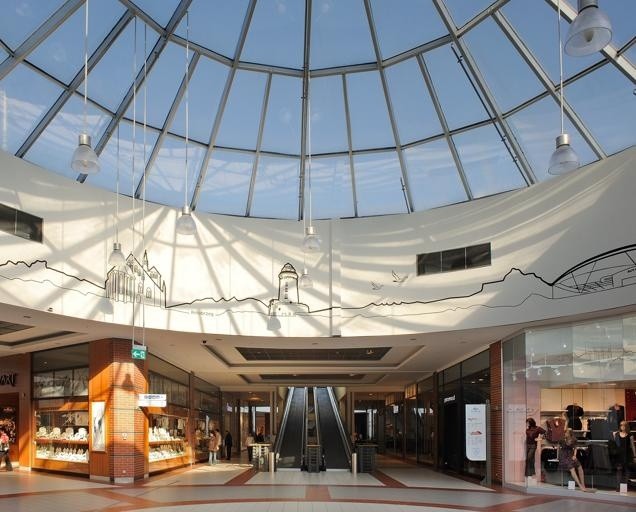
33 432 89 475
149 437 188 474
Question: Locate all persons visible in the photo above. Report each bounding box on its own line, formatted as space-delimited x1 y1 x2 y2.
207 428 233 467
525 418 547 477
559 429 589 492
608 403 624 433
245 432 254 462
563 401 584 430
0 428 13 471
174 431 183 439
255 432 265 457
611 420 636 492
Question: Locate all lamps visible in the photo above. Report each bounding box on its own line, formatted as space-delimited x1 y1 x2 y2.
175 11 197 236
71 0 101 176
299 65 324 256
296 252 313 290
108 115 127 265
549 1 579 176
562 0 614 56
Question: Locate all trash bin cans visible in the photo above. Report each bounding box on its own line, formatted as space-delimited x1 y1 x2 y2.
352 453 358 475
269 452 274 473
